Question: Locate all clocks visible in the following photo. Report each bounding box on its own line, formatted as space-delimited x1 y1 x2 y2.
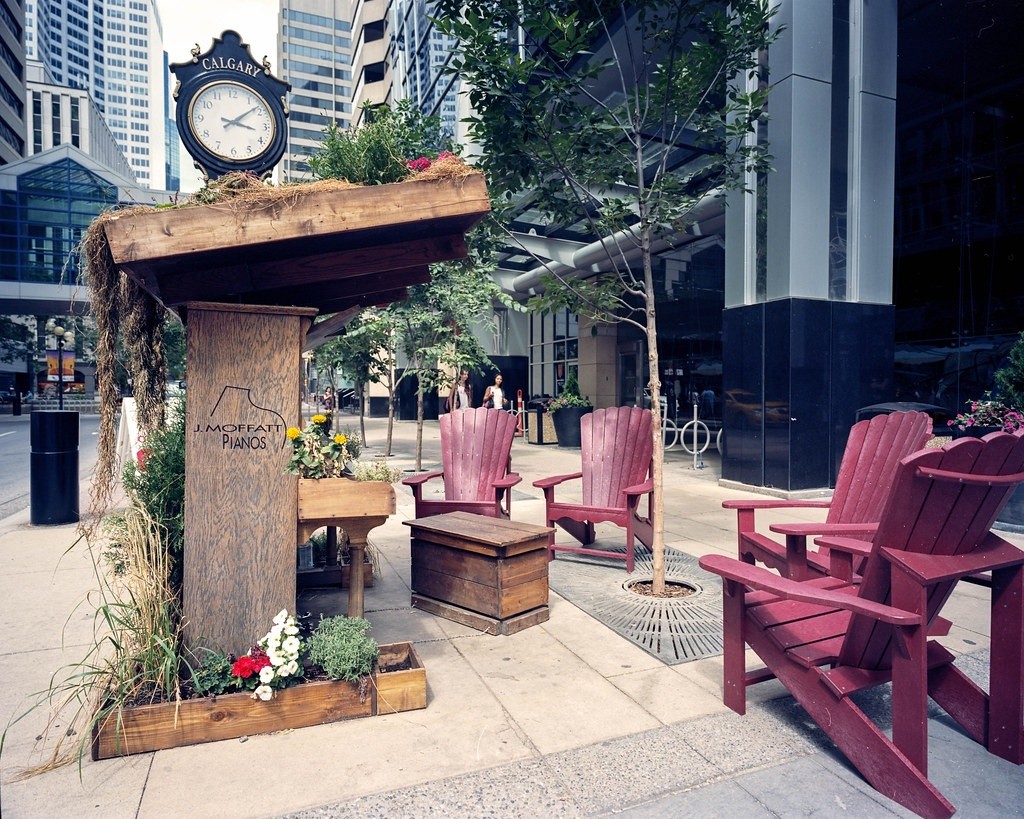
167 28 291 188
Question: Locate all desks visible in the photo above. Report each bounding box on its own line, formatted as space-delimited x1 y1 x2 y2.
402 511 558 635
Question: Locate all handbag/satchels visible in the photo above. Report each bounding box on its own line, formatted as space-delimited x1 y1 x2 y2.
443 391 461 413
321 398 327 405
481 387 494 409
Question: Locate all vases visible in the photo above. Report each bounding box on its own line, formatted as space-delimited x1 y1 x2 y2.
552 406 594 447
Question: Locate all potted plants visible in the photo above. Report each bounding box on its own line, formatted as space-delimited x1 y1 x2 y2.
0 415 426 802
973 323 1024 530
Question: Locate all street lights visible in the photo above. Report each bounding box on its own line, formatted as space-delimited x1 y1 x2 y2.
53 326 72 410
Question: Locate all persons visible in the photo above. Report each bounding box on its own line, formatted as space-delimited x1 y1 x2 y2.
25 391 33 400
684 385 716 420
483 373 508 409
448 369 473 411
323 385 335 431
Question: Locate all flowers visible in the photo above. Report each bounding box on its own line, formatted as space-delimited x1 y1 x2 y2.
230 609 301 707
545 390 594 419
286 415 364 481
945 399 1024 439
406 148 459 176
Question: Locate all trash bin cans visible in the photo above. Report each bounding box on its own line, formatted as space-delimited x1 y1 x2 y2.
30 410 80 527
527 398 559 445
854 401 953 450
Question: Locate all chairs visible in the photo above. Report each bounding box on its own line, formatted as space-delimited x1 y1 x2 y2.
699 420 1024 818
401 408 517 520
534 406 654 573
724 411 935 584
